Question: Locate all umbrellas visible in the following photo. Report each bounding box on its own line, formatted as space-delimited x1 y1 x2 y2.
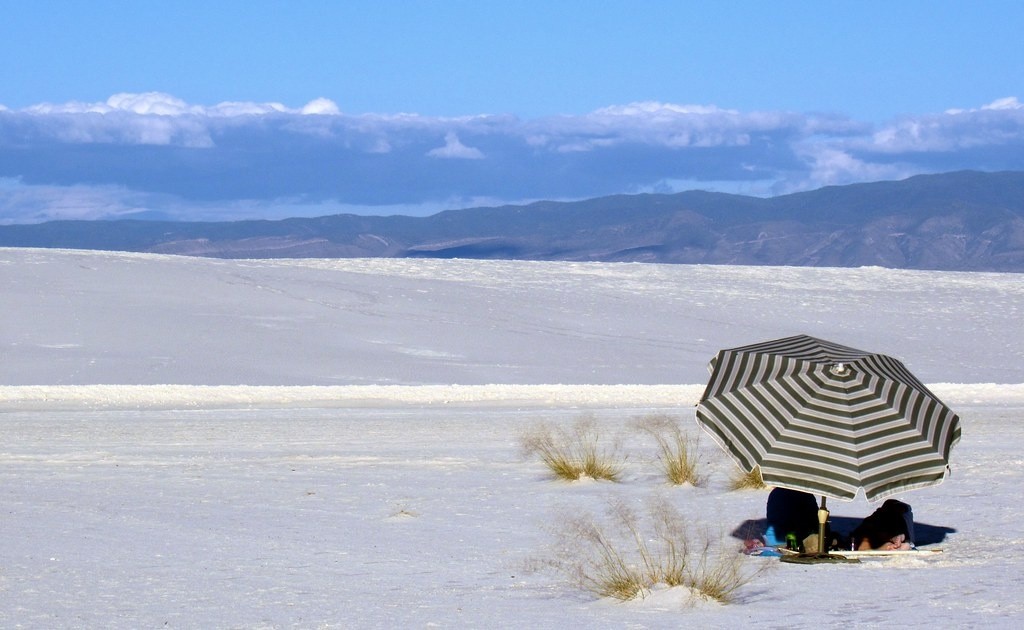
694 334 962 565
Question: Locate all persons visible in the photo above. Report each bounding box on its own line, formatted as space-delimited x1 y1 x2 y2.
766 487 820 545
874 499 914 550
